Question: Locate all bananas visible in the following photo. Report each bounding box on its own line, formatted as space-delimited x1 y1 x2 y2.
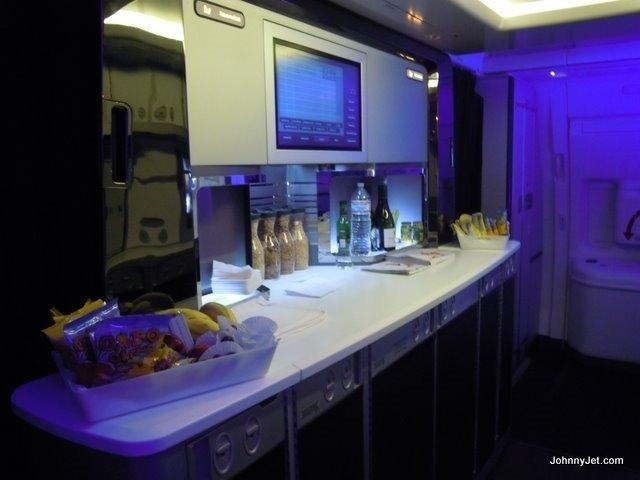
149 300 239 337
452 212 507 236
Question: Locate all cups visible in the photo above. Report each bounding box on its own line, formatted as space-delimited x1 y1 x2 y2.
271 177 296 212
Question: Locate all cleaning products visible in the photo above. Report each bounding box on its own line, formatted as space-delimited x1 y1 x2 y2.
349 182 371 255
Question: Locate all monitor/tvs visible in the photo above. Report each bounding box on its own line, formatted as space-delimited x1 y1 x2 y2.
264 20 368 165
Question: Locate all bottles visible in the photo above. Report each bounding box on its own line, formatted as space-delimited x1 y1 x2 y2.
245 208 310 280
400 220 424 244
336 182 395 257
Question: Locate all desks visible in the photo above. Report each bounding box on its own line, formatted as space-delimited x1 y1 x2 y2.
9 233 521 479
